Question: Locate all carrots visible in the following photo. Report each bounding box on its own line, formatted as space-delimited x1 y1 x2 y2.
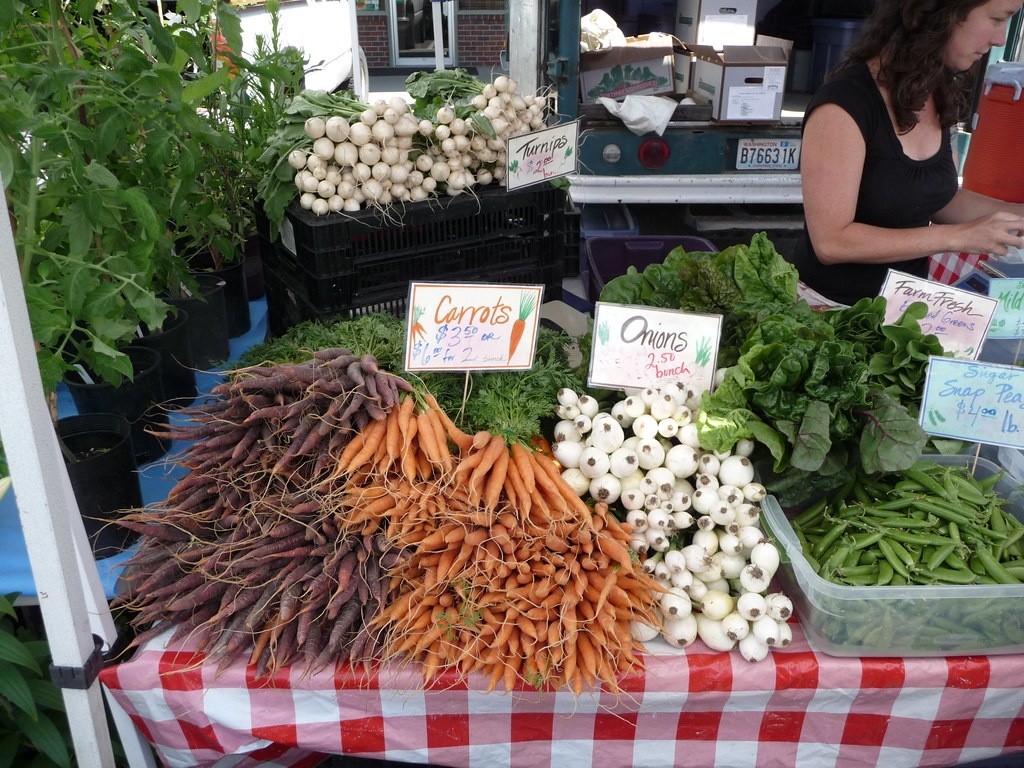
85 317 417 681
336 329 670 695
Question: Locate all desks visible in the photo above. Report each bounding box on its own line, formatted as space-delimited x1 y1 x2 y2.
0 295 269 611
99 612 1024 768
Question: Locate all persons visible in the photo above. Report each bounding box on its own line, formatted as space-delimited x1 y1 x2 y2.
794 0 1022 311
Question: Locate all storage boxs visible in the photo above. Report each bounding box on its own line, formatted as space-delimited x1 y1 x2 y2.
687 33 794 123
563 193 582 279
673 45 724 97
584 233 720 303
254 184 567 341
579 30 686 105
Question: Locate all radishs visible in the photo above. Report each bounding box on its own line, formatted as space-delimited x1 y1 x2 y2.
289 75 553 217
551 381 795 663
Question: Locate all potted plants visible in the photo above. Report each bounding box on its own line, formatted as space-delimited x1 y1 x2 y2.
1 0 262 561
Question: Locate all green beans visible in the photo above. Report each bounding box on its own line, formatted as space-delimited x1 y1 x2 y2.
789 464 1024 586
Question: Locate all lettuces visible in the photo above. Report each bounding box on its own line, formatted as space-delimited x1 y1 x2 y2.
600 231 945 506
